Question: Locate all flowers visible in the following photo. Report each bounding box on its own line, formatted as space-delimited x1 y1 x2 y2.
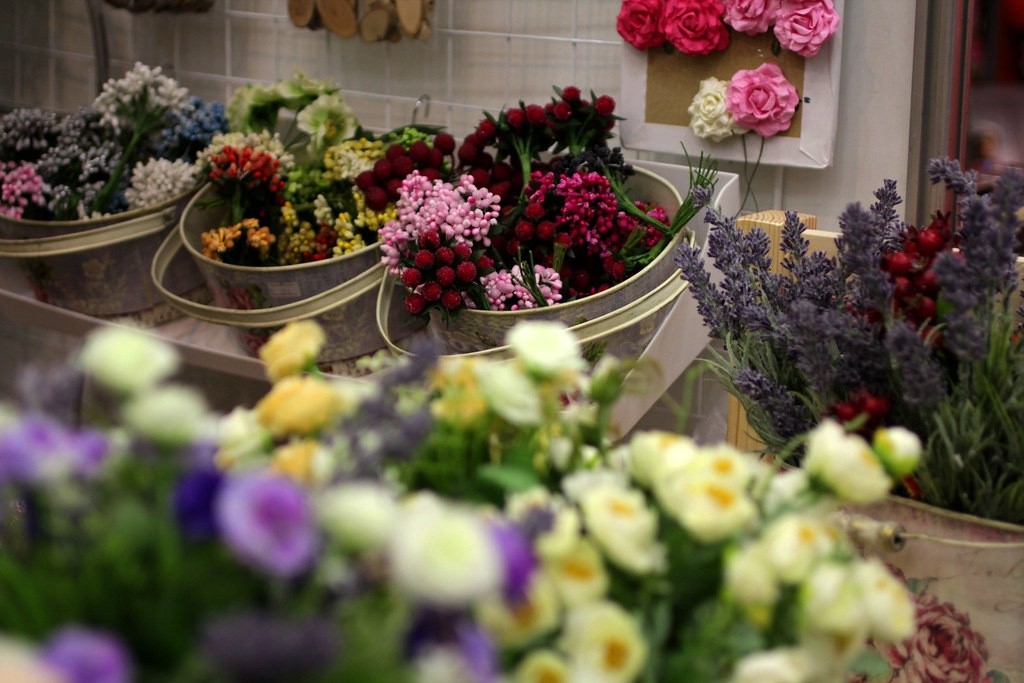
0 0 1024 683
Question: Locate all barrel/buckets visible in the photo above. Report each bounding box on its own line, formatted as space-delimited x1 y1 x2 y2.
377 157 698 372
151 175 426 362
0 181 204 318
